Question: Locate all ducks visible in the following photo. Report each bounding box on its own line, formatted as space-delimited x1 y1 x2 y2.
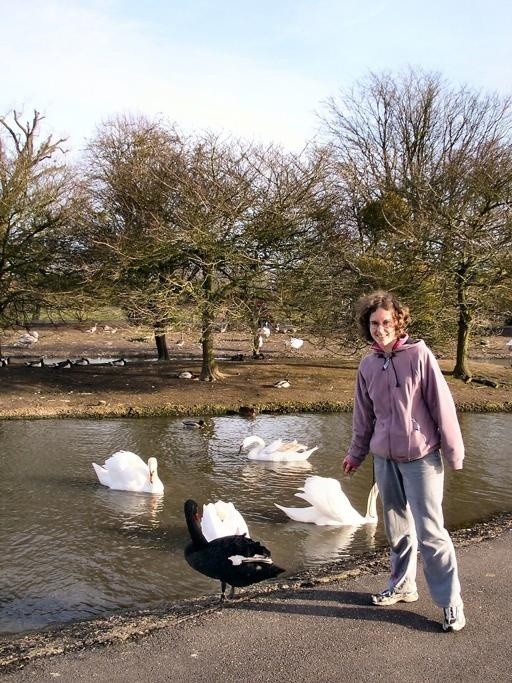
104 357 126 367
84 321 116 335
23 355 90 369
173 371 195 379
182 420 208 429
0 356 10 368
272 379 292 388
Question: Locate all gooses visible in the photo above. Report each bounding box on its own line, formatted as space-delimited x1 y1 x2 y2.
175 329 185 350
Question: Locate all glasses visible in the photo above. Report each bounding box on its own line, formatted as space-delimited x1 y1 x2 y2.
366 318 395 331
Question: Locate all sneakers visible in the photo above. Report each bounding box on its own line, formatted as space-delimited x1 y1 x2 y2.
370 589 420 609
442 598 468 634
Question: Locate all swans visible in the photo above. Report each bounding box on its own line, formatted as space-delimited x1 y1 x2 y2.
237 435 320 462
19 330 39 349
183 499 286 605
91 449 164 494
272 474 381 526
200 499 252 543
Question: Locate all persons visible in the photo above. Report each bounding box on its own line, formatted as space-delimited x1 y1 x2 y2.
340 290 468 633
